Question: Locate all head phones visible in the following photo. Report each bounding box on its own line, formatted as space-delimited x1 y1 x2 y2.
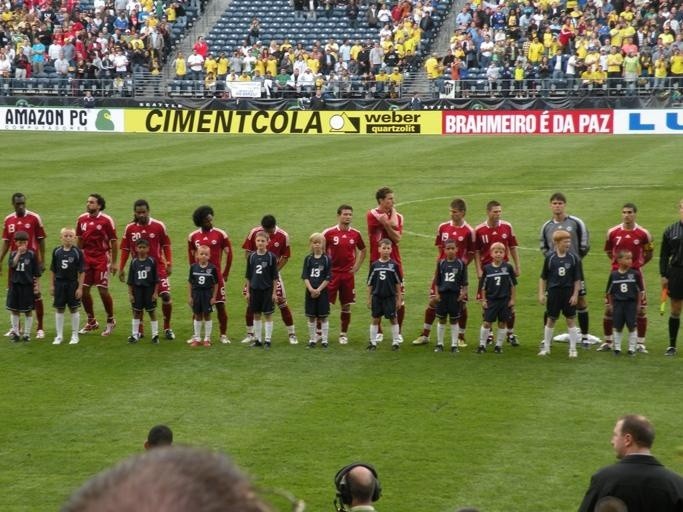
334 463 381 504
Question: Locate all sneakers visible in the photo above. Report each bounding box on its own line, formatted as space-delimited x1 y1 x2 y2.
3 318 677 356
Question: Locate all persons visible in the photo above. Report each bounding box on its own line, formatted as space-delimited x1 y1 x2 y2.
204 0 435 98
336 462 379 511
474 200 521 354
0 1 207 99
50 194 117 344
367 187 406 350
0 193 48 344
145 423 173 450
409 89 424 110
597 204 653 356
241 212 298 347
575 413 683 512
592 493 628 512
120 199 177 344
58 446 272 512
80 90 96 109
185 205 233 346
413 199 474 353
309 89 325 112
426 1 679 99
534 193 595 356
659 199 683 357
302 204 366 349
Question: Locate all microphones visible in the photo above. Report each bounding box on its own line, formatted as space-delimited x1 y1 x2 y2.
334 491 341 511
